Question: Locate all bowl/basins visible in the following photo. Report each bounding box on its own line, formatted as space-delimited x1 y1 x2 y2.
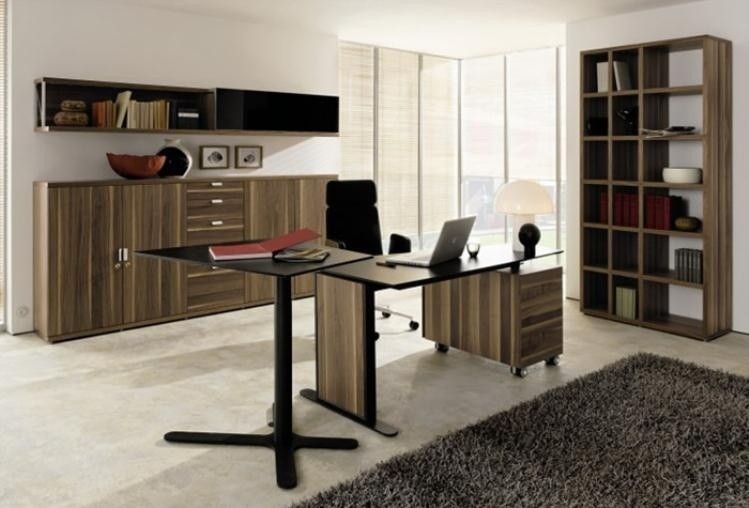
662 167 702 183
674 217 701 231
106 152 167 180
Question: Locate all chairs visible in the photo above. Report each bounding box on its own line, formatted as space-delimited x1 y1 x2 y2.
324 179 418 340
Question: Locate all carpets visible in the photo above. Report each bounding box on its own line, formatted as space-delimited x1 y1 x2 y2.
287 351 749 508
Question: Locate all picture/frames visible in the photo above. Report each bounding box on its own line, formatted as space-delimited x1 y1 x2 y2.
235 144 262 169
200 145 229 169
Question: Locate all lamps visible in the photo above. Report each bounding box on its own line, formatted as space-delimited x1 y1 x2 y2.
493 178 555 252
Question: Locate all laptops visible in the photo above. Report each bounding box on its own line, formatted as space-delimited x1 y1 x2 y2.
386 216 476 267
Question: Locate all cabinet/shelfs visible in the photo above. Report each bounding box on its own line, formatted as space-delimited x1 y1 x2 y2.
187 176 246 319
33 77 215 135
211 87 339 137
246 174 339 308
579 34 733 342
33 178 187 342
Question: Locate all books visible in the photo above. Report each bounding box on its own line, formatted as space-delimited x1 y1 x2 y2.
674 248 703 281
613 60 633 91
274 244 330 263
611 284 636 320
92 91 175 133
639 127 700 140
663 125 696 132
596 61 609 92
595 189 681 229
206 225 323 262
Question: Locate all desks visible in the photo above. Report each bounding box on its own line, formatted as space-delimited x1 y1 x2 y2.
131 237 375 490
300 247 565 437
